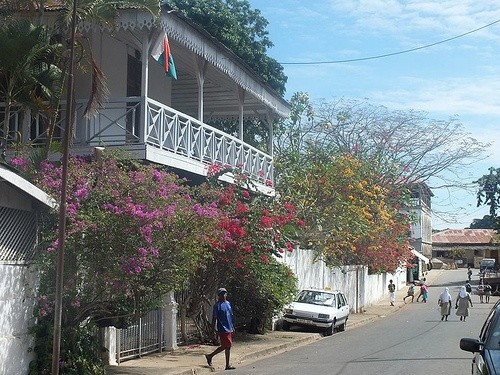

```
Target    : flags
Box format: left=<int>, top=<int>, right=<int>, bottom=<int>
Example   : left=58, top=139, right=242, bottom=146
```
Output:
left=151, top=29, right=177, bottom=80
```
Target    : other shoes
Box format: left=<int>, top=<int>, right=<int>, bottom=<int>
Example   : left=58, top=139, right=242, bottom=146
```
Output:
left=205, top=354, right=214, bottom=366
left=225, top=364, right=237, bottom=370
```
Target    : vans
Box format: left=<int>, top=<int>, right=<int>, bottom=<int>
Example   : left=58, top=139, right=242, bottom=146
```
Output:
left=478, top=258, right=496, bottom=274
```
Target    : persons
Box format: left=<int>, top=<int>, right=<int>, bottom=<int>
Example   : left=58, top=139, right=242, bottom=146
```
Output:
left=403, top=278, right=428, bottom=303
left=205, top=287, right=237, bottom=370
left=465, top=266, right=492, bottom=304
left=438, top=286, right=473, bottom=321
left=388, top=280, right=395, bottom=307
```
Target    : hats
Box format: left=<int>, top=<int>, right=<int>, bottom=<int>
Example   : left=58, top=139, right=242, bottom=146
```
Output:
left=217, top=288, right=228, bottom=295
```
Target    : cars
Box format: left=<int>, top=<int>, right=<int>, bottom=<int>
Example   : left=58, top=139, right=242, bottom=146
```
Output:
left=282, top=288, right=349, bottom=336
left=460, top=298, right=499, bottom=375
